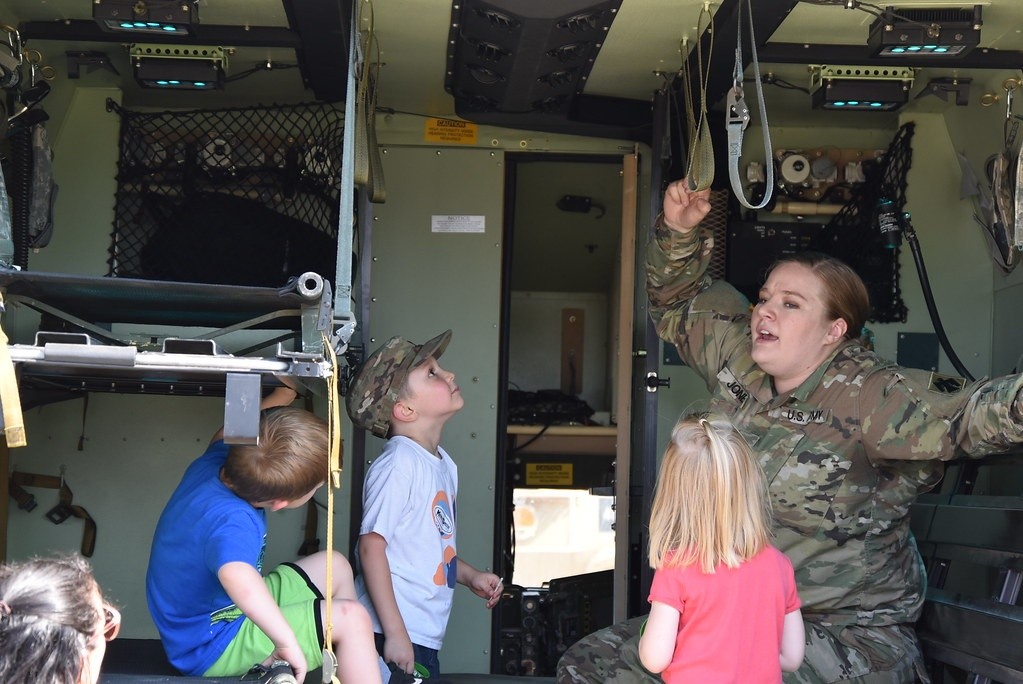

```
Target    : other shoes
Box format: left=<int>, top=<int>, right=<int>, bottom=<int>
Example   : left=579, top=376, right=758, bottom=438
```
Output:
left=264, top=661, right=299, bottom=684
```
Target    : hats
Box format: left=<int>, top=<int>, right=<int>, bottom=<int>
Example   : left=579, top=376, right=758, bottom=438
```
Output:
left=345, top=329, right=454, bottom=439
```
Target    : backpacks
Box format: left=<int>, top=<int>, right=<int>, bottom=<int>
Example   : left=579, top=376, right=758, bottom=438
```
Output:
left=970, top=112, right=1023, bottom=273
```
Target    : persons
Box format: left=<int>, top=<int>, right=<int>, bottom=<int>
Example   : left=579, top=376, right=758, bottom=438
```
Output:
left=0, top=552, right=123, bottom=684
left=638, top=412, right=805, bottom=684
left=345, top=329, right=504, bottom=684
left=145, top=387, right=382, bottom=684
left=556, top=177, right=1023, bottom=684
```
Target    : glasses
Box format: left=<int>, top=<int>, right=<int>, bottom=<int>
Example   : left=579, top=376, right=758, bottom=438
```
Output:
left=85, top=603, right=122, bottom=648
